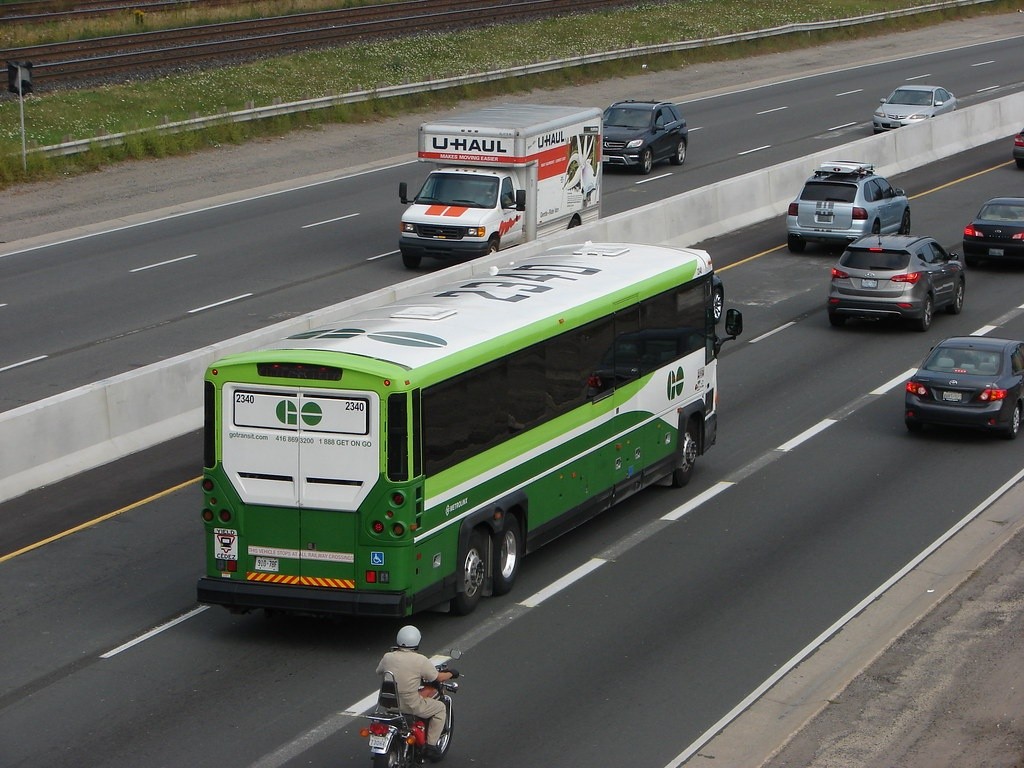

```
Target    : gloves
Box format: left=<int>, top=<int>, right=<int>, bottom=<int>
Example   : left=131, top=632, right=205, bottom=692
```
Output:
left=447, top=669, right=459, bottom=680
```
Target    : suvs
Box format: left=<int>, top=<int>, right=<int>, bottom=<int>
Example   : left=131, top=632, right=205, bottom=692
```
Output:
left=786, top=160, right=911, bottom=253
left=600, top=100, right=688, bottom=174
left=826, top=233, right=965, bottom=333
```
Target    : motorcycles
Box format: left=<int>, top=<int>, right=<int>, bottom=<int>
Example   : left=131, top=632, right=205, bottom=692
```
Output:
left=360, top=648, right=465, bottom=768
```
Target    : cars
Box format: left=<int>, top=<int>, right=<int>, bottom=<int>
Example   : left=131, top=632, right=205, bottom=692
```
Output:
left=906, top=336, right=1024, bottom=438
left=590, top=327, right=706, bottom=394
left=1013, top=128, right=1024, bottom=168
left=963, top=196, right=1024, bottom=269
left=872, top=84, right=957, bottom=134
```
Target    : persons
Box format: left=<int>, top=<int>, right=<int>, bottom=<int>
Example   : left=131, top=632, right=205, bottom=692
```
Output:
left=488, top=185, right=512, bottom=205
left=375, top=625, right=459, bottom=759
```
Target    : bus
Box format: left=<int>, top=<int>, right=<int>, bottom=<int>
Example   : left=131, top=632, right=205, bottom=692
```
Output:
left=196, top=239, right=741, bottom=618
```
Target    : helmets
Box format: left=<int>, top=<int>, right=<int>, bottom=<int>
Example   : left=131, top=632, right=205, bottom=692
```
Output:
left=396, top=624, right=422, bottom=648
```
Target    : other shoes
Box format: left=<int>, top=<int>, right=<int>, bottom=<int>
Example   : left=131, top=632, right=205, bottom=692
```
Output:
left=422, top=745, right=442, bottom=758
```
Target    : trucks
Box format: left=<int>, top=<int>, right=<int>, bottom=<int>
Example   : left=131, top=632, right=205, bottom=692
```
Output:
left=399, top=101, right=602, bottom=269
